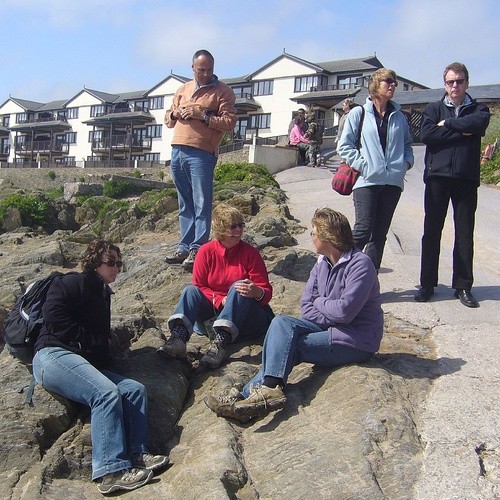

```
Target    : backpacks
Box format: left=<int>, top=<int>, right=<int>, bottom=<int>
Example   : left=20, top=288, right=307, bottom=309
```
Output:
left=2, top=271, right=87, bottom=402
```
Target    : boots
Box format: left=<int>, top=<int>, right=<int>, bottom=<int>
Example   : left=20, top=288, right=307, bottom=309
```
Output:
left=155, top=323, right=191, bottom=361
left=199, top=330, right=231, bottom=369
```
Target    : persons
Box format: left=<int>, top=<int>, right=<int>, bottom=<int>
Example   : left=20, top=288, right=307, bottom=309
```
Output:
left=234, top=208, right=383, bottom=415
left=32, top=239, right=170, bottom=493
left=336, top=68, right=414, bottom=276
left=288, top=108, right=323, bottom=167
left=334, top=99, right=354, bottom=163
left=163, top=202, right=275, bottom=368
left=164, top=50, right=237, bottom=268
left=415, top=62, right=490, bottom=308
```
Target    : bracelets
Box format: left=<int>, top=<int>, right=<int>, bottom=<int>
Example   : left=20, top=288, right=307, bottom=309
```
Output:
left=255, top=286, right=265, bottom=301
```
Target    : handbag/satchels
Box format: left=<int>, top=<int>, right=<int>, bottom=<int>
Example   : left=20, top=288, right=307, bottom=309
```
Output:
left=332, top=161, right=362, bottom=195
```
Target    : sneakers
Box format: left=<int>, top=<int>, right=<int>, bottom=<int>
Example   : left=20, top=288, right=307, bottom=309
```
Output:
left=97, top=466, right=154, bottom=495
left=164, top=251, right=186, bottom=264
left=205, top=387, right=259, bottom=421
left=124, top=452, right=170, bottom=471
left=181, top=248, right=199, bottom=269
left=233, top=382, right=287, bottom=415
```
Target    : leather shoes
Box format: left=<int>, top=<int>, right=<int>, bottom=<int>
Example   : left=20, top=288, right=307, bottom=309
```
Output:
left=414, top=287, right=435, bottom=302
left=454, top=288, right=478, bottom=307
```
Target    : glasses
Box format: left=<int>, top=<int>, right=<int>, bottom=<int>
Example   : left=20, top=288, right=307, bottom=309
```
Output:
left=380, top=78, right=398, bottom=87
left=445, top=79, right=466, bottom=86
left=310, top=231, right=316, bottom=237
left=101, top=260, right=123, bottom=267
left=225, top=222, right=245, bottom=229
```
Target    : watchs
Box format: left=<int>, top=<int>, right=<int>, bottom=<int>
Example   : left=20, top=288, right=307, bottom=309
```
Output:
left=200, top=111, right=210, bottom=123
